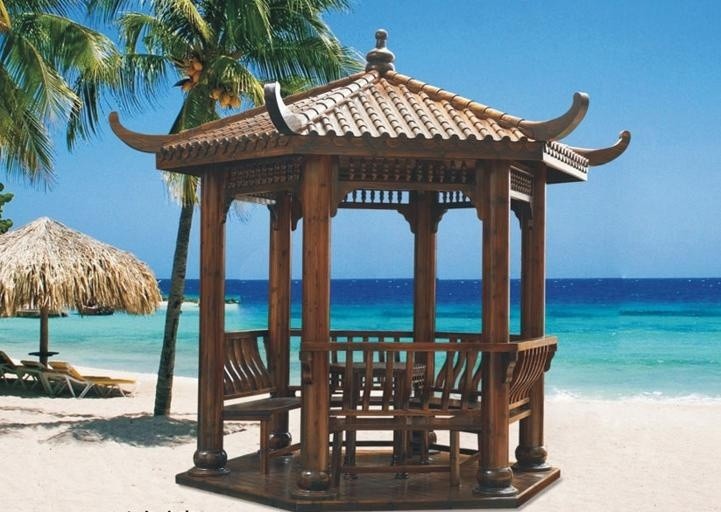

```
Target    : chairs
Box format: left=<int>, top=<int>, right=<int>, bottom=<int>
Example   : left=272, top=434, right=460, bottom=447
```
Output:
left=0, top=351, right=136, bottom=397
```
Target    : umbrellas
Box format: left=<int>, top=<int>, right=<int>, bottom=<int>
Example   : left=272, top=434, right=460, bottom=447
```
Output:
left=0, top=215, right=164, bottom=370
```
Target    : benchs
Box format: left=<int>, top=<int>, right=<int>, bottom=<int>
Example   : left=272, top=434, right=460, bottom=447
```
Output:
left=223, top=331, right=557, bottom=491
